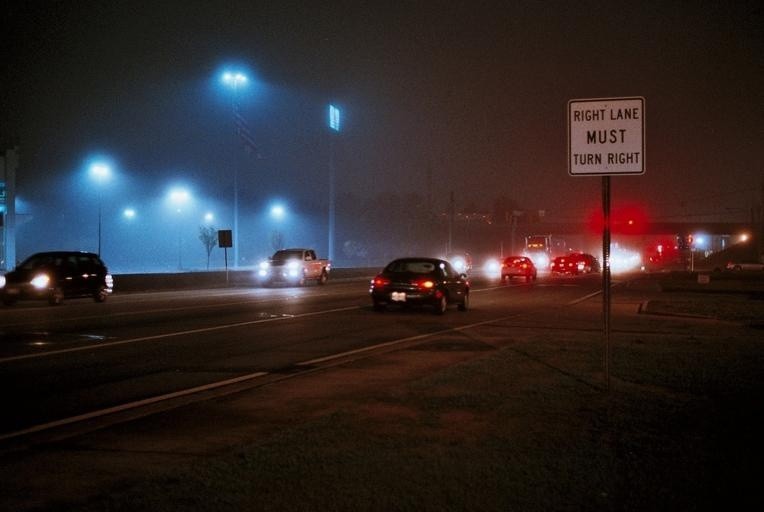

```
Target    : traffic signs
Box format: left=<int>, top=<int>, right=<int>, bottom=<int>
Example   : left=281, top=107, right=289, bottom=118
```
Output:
left=567, top=95, right=646, bottom=177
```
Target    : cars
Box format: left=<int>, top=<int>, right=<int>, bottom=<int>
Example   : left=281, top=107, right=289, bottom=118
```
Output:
left=0, top=251, right=113, bottom=307
left=724, top=256, right=764, bottom=272
left=369, top=253, right=600, bottom=315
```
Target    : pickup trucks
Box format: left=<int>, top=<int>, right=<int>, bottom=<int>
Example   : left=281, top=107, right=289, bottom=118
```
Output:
left=258, top=248, right=332, bottom=286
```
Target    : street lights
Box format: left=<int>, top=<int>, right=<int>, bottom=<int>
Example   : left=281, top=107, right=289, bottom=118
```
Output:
left=89, top=164, right=214, bottom=270
left=272, top=208, right=282, bottom=248
left=220, top=69, right=249, bottom=264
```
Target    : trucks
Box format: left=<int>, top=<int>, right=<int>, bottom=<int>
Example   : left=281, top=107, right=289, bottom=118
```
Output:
left=525, top=234, right=566, bottom=268
